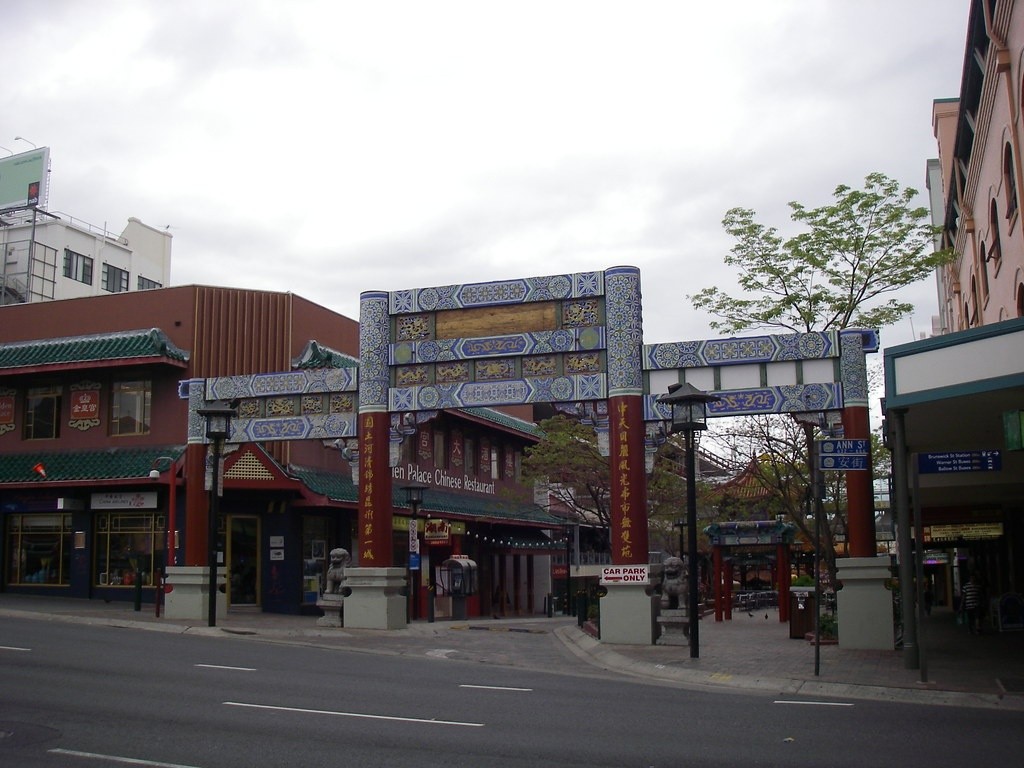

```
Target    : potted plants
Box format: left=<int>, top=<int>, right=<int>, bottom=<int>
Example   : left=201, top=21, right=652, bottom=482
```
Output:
left=819, top=613, right=837, bottom=641
left=789, top=575, right=816, bottom=592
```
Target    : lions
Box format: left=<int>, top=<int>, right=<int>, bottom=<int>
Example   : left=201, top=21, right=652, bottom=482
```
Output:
left=660, top=556, right=687, bottom=610
left=324, top=548, right=352, bottom=595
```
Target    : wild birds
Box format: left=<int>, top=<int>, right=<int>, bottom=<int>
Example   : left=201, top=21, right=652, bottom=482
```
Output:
left=748, top=612, right=755, bottom=619
left=765, top=613, right=768, bottom=620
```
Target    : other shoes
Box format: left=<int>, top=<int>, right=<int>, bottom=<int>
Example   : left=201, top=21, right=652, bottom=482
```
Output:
left=968, top=629, right=972, bottom=634
left=976, top=629, right=982, bottom=634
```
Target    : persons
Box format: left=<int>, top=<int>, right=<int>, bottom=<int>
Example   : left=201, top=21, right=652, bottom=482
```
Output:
left=923, top=578, right=936, bottom=618
left=959, top=572, right=986, bottom=641
